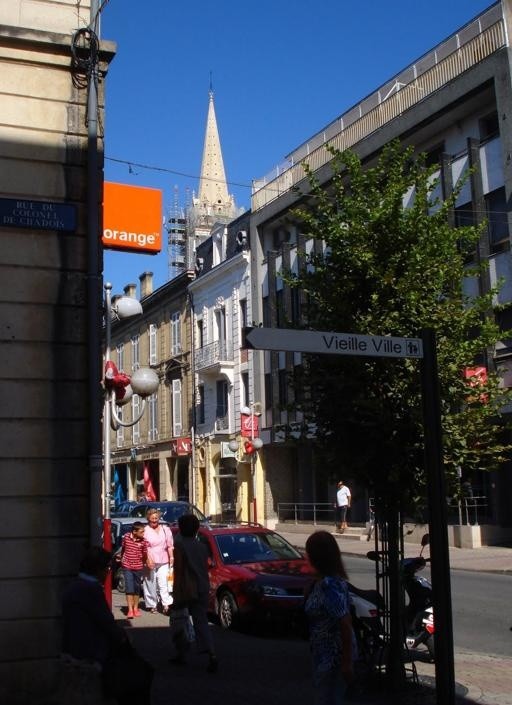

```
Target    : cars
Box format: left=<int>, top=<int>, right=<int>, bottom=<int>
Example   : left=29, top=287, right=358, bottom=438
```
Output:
left=100, top=498, right=328, bottom=637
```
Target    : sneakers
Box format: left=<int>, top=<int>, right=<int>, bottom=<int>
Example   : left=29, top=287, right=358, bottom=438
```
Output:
left=134, top=608, right=142, bottom=616
left=163, top=608, right=169, bottom=615
left=151, top=608, right=157, bottom=613
left=127, top=610, right=134, bottom=618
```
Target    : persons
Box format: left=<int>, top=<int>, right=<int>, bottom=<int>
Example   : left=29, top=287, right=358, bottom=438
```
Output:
left=59, top=544, right=156, bottom=705
left=143, top=508, right=176, bottom=616
left=120, top=521, right=149, bottom=619
left=304, top=531, right=369, bottom=705
left=332, top=480, right=351, bottom=533
left=171, top=514, right=220, bottom=676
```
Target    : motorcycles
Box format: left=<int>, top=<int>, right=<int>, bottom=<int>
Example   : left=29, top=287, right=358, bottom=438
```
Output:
left=346, top=530, right=435, bottom=667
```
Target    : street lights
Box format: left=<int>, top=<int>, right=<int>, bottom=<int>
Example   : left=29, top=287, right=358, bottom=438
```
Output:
left=102, top=281, right=165, bottom=554
left=227, top=400, right=269, bottom=525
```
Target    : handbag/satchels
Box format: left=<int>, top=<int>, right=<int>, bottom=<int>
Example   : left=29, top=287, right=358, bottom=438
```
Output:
left=350, top=604, right=378, bottom=675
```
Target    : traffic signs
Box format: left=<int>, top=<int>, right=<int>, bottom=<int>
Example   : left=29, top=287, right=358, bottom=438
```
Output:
left=242, top=327, right=425, bottom=360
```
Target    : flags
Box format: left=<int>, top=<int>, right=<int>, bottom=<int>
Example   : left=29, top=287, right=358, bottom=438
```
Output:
left=143, top=461, right=157, bottom=502
left=113, top=465, right=127, bottom=512
